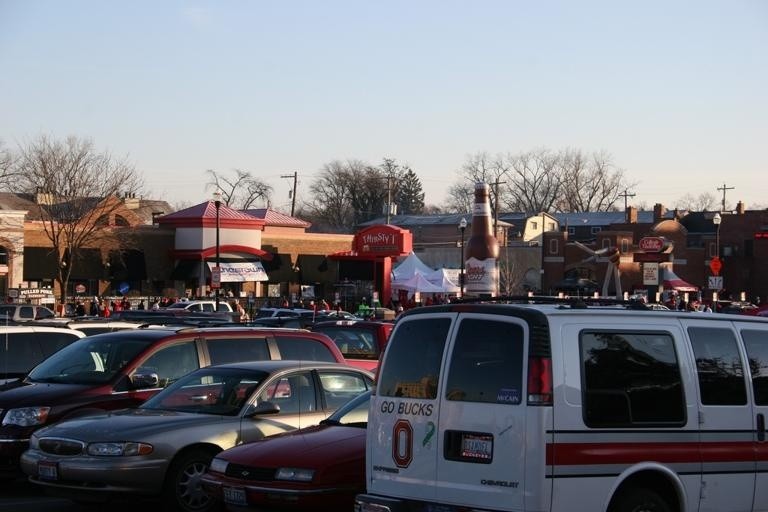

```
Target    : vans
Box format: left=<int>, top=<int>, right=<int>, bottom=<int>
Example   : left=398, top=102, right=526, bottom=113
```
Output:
left=0, top=323, right=348, bottom=461
left=333, top=283, right=358, bottom=299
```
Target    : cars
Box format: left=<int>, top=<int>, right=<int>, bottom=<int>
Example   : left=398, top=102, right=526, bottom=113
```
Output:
left=200, top=388, right=372, bottom=511
left=0, top=299, right=767, bottom=370
left=552, top=279, right=599, bottom=298
left=20, top=359, right=377, bottom=511
left=0, top=321, right=105, bottom=387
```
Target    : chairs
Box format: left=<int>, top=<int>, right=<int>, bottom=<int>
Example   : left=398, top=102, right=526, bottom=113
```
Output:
left=280, top=384, right=313, bottom=414
left=235, top=383, right=266, bottom=412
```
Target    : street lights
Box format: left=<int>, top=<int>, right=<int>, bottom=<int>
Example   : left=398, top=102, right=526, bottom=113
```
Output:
left=214, top=187, right=222, bottom=312
left=713, top=212, right=722, bottom=313
left=106, top=262, right=110, bottom=297
left=293, top=266, right=302, bottom=301
left=460, top=218, right=467, bottom=299
left=60, top=262, right=66, bottom=304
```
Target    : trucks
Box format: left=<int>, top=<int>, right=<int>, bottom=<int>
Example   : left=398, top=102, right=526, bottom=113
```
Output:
left=353, top=293, right=767, bottom=511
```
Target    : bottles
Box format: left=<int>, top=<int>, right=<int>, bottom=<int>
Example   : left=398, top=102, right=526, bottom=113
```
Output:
left=460, top=183, right=503, bottom=300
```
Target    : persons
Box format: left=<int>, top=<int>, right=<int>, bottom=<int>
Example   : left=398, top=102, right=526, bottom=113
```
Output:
left=25, top=294, right=31, bottom=305
left=395, top=292, right=457, bottom=313
left=227, top=288, right=342, bottom=323
left=664, top=294, right=712, bottom=314
left=74, top=291, right=191, bottom=318
